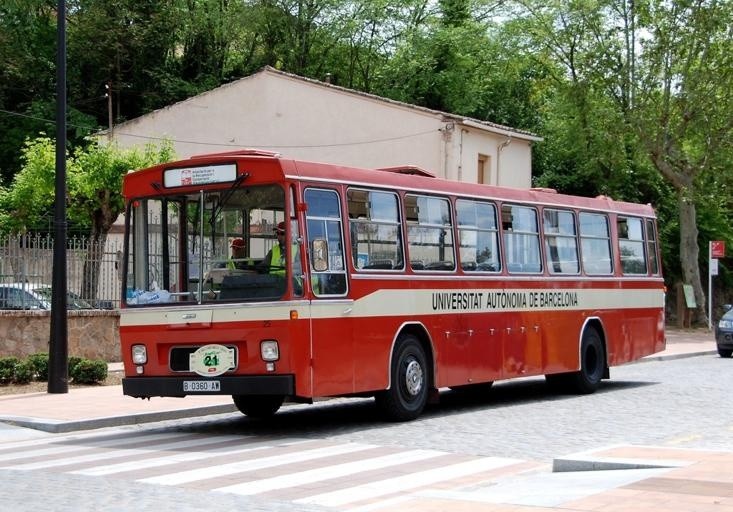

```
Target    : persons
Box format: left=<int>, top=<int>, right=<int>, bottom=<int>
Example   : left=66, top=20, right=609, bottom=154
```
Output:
left=258, top=221, right=320, bottom=295
left=211, top=238, right=254, bottom=289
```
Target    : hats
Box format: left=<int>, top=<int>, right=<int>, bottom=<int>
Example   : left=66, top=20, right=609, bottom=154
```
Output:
left=272, top=222, right=285, bottom=232
left=230, top=238, right=245, bottom=249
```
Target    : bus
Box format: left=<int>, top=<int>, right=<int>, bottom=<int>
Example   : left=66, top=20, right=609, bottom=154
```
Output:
left=118, top=149, right=669, bottom=420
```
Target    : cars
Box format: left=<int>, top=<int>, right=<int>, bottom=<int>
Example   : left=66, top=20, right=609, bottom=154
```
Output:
left=714, top=304, right=733, bottom=357
left=0, top=283, right=106, bottom=310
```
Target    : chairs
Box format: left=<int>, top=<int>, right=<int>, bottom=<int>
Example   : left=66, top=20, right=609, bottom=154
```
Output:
left=363, top=257, right=653, bottom=275
